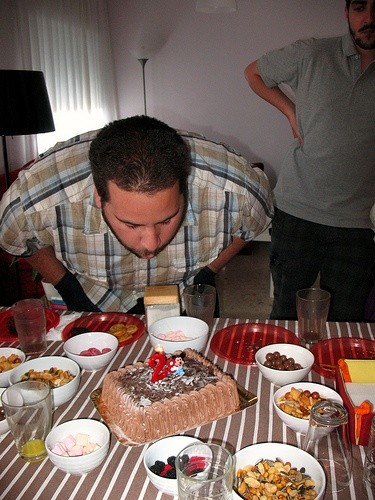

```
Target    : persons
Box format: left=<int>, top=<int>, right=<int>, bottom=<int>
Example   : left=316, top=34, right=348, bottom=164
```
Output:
left=244, top=0, right=375, bottom=323
left=0, top=114, right=276, bottom=317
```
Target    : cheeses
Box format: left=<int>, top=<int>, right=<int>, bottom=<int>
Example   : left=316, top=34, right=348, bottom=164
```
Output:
left=52, top=434, right=100, bottom=457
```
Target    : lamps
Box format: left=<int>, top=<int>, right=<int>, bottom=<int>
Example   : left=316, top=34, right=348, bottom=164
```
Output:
left=0, top=69, right=55, bottom=173
left=126, top=21, right=164, bottom=116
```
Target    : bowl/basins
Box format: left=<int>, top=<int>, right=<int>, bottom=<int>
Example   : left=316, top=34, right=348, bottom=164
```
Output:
left=254, top=342, right=315, bottom=386
left=142, top=437, right=214, bottom=495
left=44, top=419, right=111, bottom=476
left=0, top=327, right=120, bottom=438
left=148, top=316, right=209, bottom=353
left=222, top=442, right=326, bottom=500
left=272, top=381, right=344, bottom=436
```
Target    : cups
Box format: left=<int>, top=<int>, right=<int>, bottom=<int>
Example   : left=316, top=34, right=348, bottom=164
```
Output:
left=294, top=289, right=331, bottom=343
left=3, top=380, right=54, bottom=463
left=175, top=442, right=234, bottom=500
left=12, top=297, right=47, bottom=355
left=182, top=285, right=216, bottom=333
left=303, top=403, right=353, bottom=494
left=363, top=418, right=375, bottom=488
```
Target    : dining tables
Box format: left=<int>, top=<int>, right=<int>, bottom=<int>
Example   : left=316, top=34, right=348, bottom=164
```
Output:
left=0, top=306, right=375, bottom=500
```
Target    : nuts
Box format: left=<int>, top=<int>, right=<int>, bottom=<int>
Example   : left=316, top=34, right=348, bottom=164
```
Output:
left=236, top=462, right=316, bottom=500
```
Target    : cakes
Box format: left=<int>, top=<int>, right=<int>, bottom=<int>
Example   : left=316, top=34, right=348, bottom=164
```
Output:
left=100, top=347, right=240, bottom=443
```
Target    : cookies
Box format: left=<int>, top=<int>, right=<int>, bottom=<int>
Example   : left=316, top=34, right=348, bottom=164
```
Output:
left=105, top=324, right=137, bottom=342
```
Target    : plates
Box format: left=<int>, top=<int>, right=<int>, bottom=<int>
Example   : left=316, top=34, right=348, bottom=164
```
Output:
left=0, top=304, right=60, bottom=344
left=63, top=312, right=145, bottom=349
left=309, top=337, right=375, bottom=379
left=209, top=323, right=300, bottom=366
